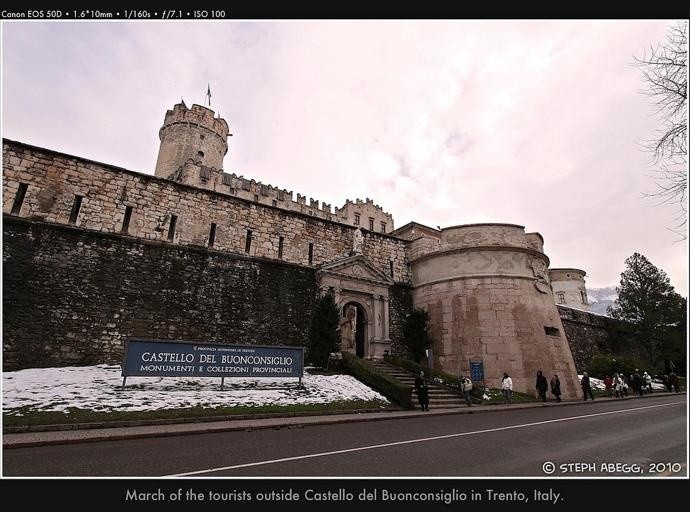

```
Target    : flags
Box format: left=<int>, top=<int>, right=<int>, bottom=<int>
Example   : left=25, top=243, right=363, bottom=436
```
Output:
left=208, top=83, right=211, bottom=106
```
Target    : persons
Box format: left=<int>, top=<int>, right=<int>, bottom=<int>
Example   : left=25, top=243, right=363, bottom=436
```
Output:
left=501, top=373, right=513, bottom=406
left=353, top=226, right=365, bottom=253
left=415, top=370, right=429, bottom=411
left=604, top=369, right=681, bottom=399
left=536, top=370, right=548, bottom=404
left=550, top=375, right=561, bottom=403
left=460, top=374, right=473, bottom=405
left=581, top=372, right=594, bottom=401
